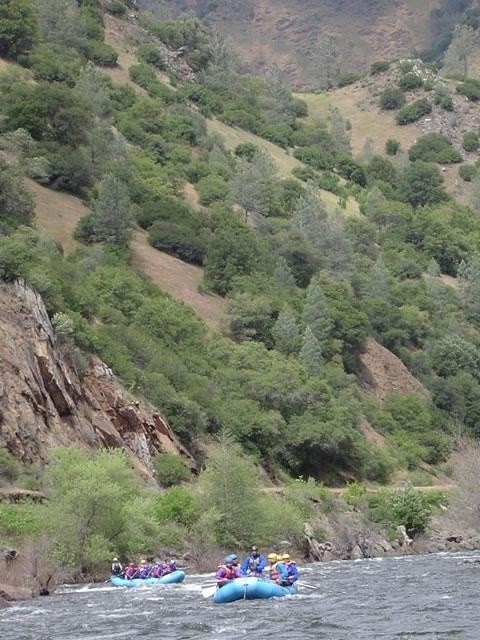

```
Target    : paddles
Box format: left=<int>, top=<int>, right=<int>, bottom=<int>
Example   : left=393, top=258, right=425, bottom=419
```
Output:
left=201, top=580, right=217, bottom=600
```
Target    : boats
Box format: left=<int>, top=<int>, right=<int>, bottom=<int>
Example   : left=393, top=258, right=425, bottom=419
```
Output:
left=213, top=575, right=297, bottom=603
left=110, top=569, right=185, bottom=589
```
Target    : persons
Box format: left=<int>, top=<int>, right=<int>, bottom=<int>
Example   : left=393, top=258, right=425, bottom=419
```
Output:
left=262, top=552, right=300, bottom=586
left=215, top=556, right=244, bottom=589
left=228, top=553, right=244, bottom=571
left=240, top=545, right=268, bottom=577
left=110, top=556, right=178, bottom=580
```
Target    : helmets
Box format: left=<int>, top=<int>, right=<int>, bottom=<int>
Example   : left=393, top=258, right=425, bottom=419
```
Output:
left=229, top=553, right=238, bottom=560
left=224, top=557, right=232, bottom=563
left=251, top=545, right=258, bottom=551
left=267, top=553, right=290, bottom=563
